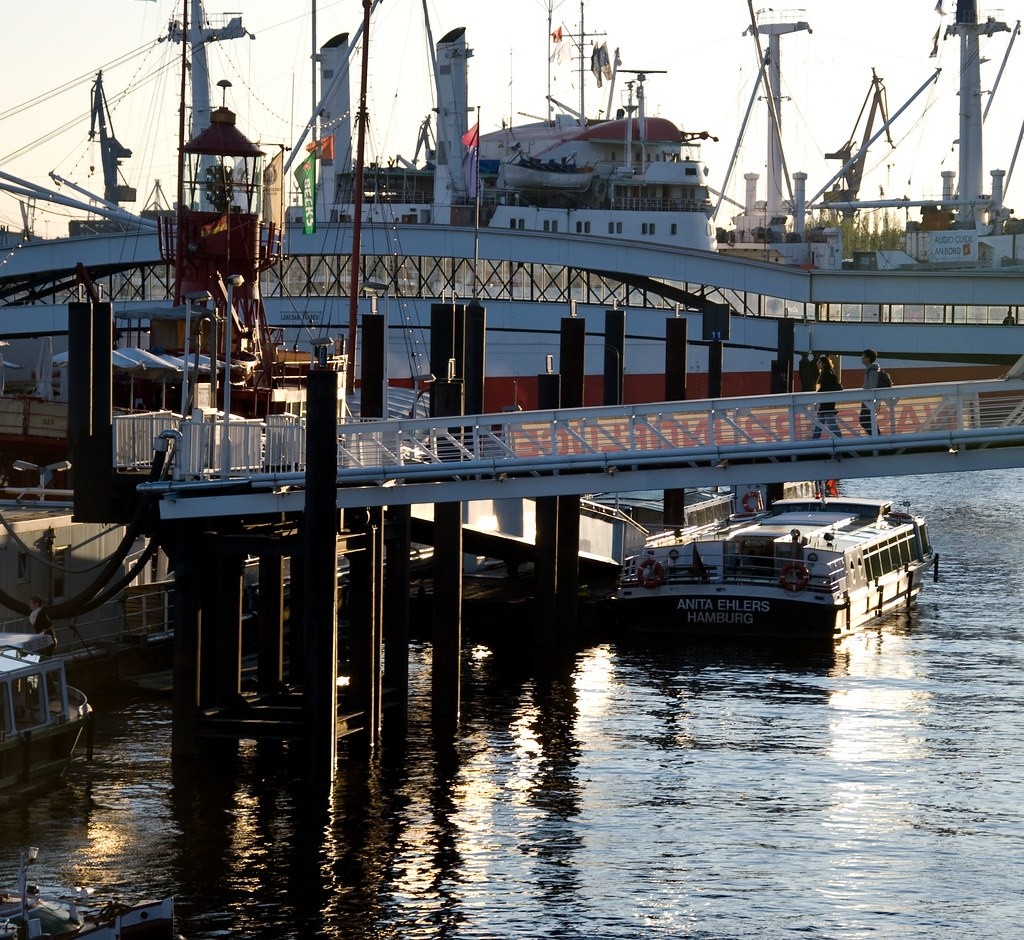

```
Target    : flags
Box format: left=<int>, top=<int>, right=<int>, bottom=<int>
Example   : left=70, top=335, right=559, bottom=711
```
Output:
left=305, top=134, right=335, bottom=160
left=294, top=149, right=316, bottom=235
left=591, top=39, right=613, bottom=88
left=264, top=149, right=286, bottom=236
left=461, top=122, right=480, bottom=148
left=548, top=26, right=575, bottom=68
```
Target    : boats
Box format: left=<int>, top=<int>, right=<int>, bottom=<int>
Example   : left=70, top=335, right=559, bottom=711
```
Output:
left=0, top=847, right=173, bottom=940
left=1, top=630, right=92, bottom=798
left=1, top=1, right=1023, bottom=460
left=617, top=492, right=939, bottom=640
left=729, top=479, right=838, bottom=513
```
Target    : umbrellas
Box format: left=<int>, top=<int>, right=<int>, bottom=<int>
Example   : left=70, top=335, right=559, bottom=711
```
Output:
left=34, top=336, right=54, bottom=400
left=50, top=344, right=244, bottom=414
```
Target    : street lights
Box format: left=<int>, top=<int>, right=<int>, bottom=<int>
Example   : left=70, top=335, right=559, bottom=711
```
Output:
left=221, top=274, right=245, bottom=474
left=180, top=290, right=212, bottom=418
left=13, top=459, right=71, bottom=502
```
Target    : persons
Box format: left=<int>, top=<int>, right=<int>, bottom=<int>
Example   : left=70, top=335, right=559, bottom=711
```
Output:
left=812, top=358, right=843, bottom=438
left=28, top=594, right=59, bottom=700
left=859, top=350, right=884, bottom=436
left=1003, top=312, right=1014, bottom=325
left=799, top=350, right=819, bottom=392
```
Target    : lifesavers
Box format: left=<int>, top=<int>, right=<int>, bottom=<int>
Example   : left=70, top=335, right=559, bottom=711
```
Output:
left=638, top=560, right=664, bottom=588
left=743, top=491, right=764, bottom=512
left=780, top=561, right=808, bottom=592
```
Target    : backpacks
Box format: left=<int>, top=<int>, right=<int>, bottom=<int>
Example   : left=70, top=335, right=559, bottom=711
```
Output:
left=867, top=368, right=892, bottom=388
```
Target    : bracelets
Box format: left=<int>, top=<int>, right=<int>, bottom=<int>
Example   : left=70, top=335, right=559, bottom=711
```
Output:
left=41, top=631, right=45, bottom=635
